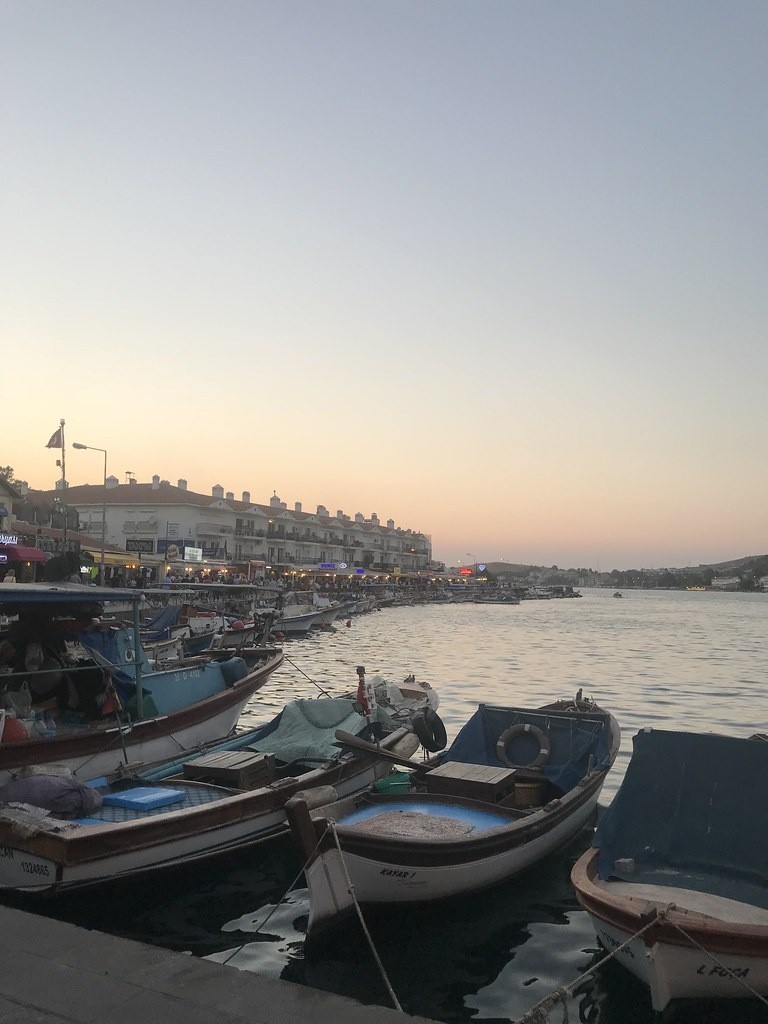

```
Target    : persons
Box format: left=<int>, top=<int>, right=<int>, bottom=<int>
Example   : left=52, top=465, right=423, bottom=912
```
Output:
left=3, top=569, right=16, bottom=583
left=70, top=570, right=431, bottom=606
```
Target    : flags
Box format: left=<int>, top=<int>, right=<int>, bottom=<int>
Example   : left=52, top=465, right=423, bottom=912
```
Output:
left=44, top=428, right=62, bottom=449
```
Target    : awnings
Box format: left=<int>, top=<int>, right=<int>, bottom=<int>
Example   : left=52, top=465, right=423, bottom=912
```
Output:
left=88, top=552, right=139, bottom=566
left=7, top=545, right=48, bottom=562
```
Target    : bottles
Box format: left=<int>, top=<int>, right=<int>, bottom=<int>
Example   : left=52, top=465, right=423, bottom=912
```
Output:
left=36, top=716, right=48, bottom=738
left=46, top=715, right=56, bottom=737
left=5, top=705, right=16, bottom=719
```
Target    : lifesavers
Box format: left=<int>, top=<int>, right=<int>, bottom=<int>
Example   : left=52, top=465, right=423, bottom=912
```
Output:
left=497, top=723, right=550, bottom=768
left=413, top=706, right=446, bottom=752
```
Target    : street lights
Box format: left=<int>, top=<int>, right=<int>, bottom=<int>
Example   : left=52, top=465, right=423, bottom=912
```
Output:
left=72, top=443, right=108, bottom=588
left=268, top=517, right=287, bottom=590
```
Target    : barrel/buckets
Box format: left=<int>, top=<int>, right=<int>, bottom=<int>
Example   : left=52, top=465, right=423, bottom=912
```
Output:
left=375, top=773, right=411, bottom=793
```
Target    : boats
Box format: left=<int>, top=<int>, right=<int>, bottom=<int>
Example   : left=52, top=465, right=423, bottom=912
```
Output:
left=283, top=687, right=620, bottom=937
left=114, top=587, right=583, bottom=664
left=568, top=726, right=768, bottom=1012
left=0, top=581, right=285, bottom=788
left=0, top=674, right=440, bottom=897
